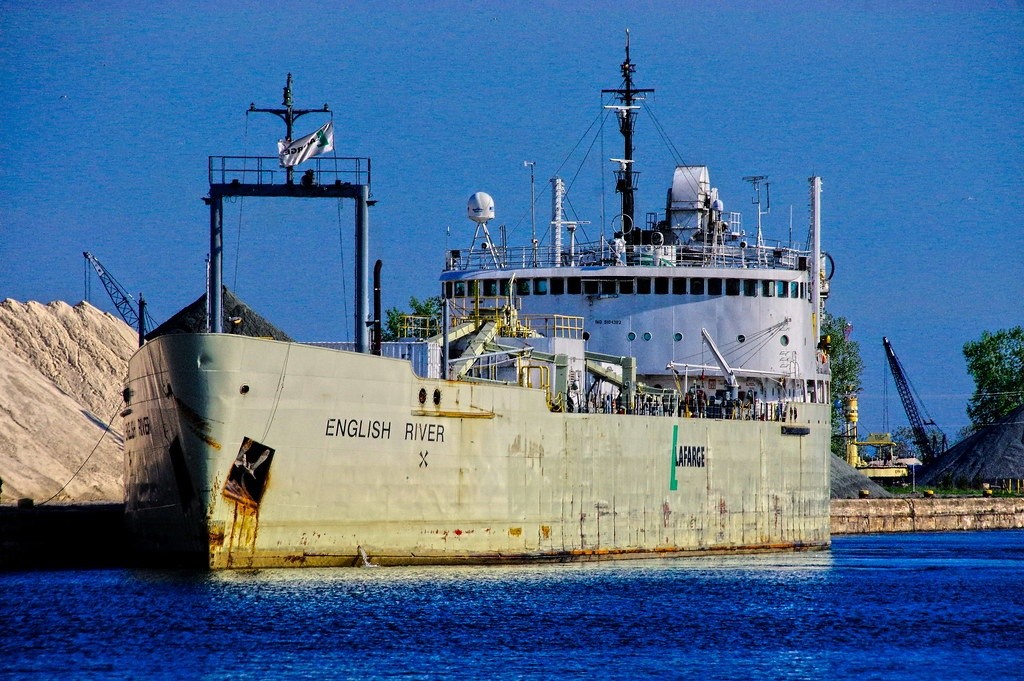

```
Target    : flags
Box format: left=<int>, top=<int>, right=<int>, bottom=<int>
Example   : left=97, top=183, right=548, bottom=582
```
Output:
left=276, top=121, right=334, bottom=168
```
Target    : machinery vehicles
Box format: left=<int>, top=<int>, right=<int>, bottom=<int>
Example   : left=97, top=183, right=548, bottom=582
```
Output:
left=83, top=252, right=159, bottom=336
left=882, top=337, right=948, bottom=464
left=838, top=384, right=909, bottom=485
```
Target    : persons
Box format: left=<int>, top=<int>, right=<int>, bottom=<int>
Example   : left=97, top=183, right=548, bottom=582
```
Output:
left=567, top=383, right=708, bottom=421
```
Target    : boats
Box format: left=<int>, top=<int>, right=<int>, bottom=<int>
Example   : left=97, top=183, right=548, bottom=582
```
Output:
left=122, top=29, right=835, bottom=572
